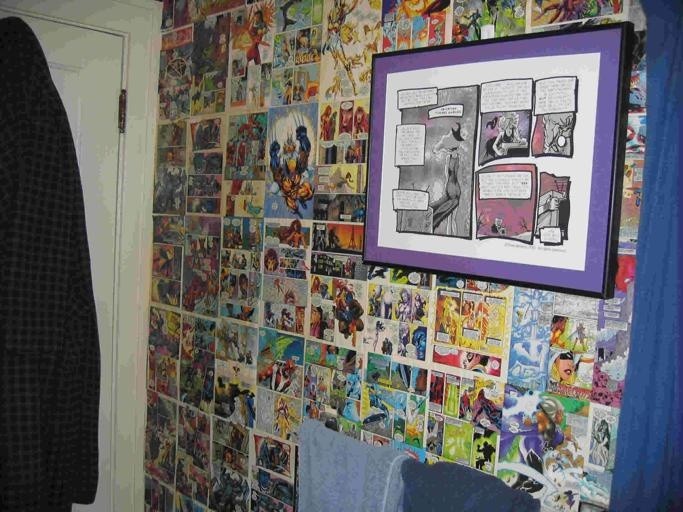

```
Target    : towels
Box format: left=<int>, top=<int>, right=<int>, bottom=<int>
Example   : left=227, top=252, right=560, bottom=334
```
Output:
left=299, top=419, right=408, bottom=512
left=401, top=459, right=540, bottom=512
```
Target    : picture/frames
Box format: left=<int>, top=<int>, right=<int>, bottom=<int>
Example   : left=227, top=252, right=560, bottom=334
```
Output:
left=363, top=22, right=634, bottom=299
